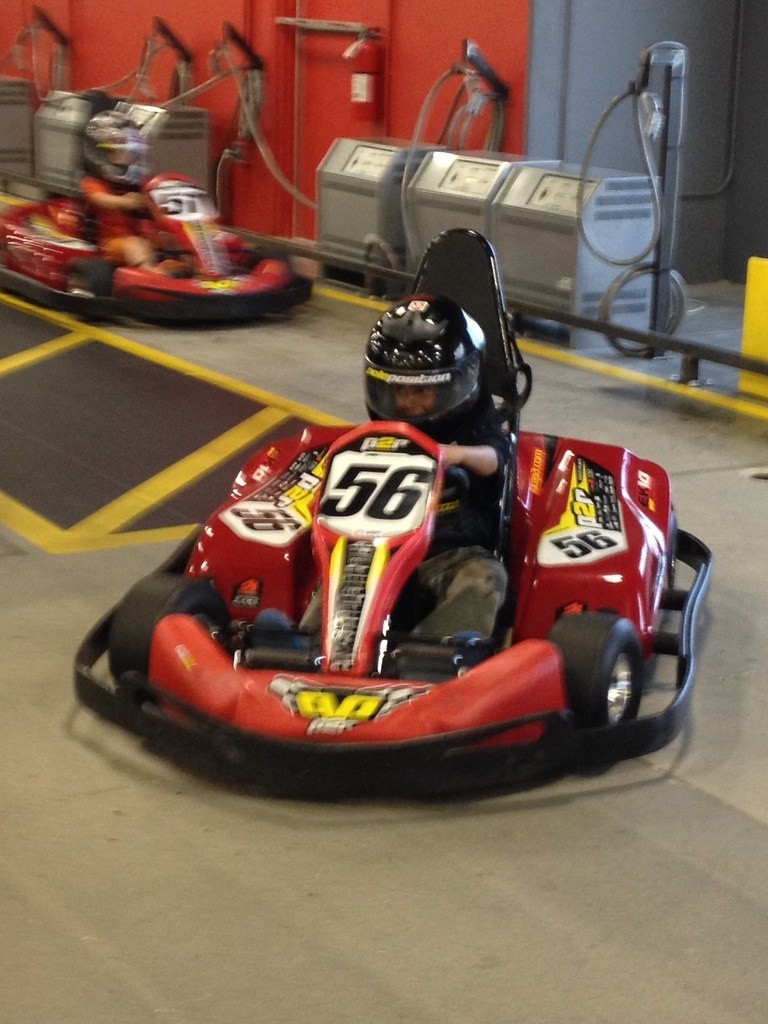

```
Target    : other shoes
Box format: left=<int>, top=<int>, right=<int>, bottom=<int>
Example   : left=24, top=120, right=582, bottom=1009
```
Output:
left=253, top=609, right=302, bottom=652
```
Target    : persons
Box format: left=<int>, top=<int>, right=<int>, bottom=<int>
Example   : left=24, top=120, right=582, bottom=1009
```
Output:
left=250, top=295, right=510, bottom=683
left=78, top=111, right=269, bottom=276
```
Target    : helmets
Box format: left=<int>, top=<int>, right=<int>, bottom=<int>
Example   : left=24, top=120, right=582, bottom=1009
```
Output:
left=362, top=292, right=487, bottom=438
left=82, top=109, right=147, bottom=187
